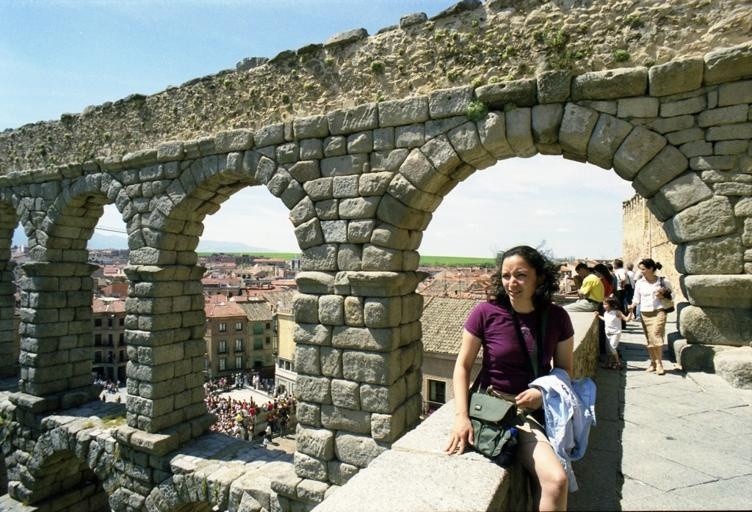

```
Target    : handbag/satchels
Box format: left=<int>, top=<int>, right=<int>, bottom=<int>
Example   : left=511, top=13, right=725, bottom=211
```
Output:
left=661, top=293, right=675, bottom=313
left=461, top=386, right=520, bottom=465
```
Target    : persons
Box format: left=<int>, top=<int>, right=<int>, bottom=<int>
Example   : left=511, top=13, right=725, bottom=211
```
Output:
left=444, top=242, right=597, bottom=512
left=201, top=368, right=296, bottom=443
left=92, top=373, right=122, bottom=404
left=562, top=256, right=675, bottom=375
